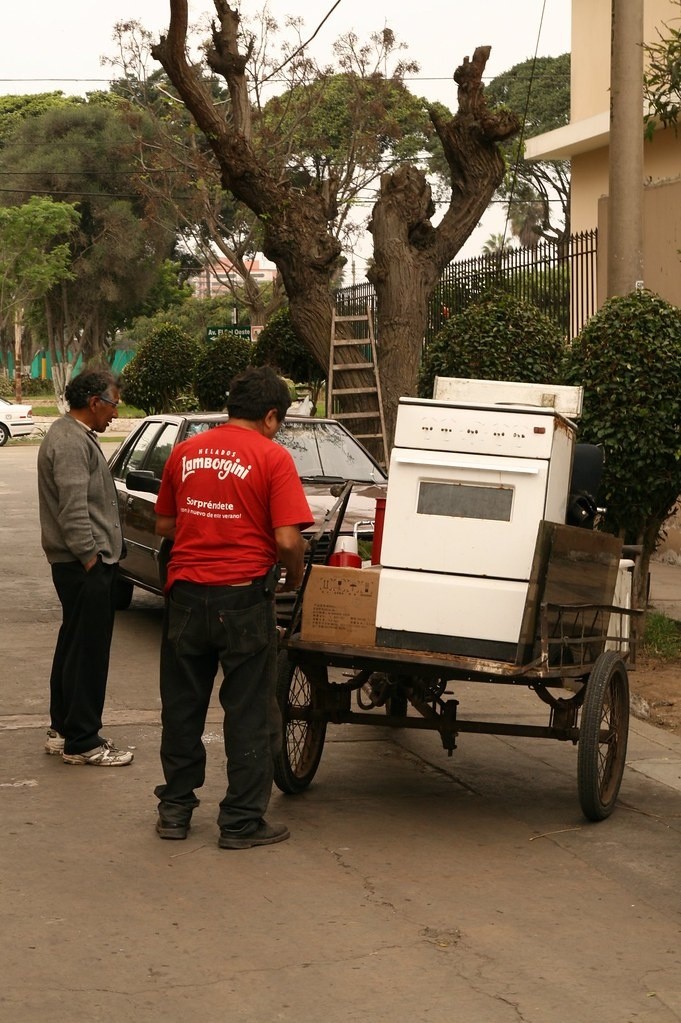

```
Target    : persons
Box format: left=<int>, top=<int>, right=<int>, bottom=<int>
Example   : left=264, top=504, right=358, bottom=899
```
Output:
left=38, top=369, right=134, bottom=767
left=154, top=369, right=316, bottom=848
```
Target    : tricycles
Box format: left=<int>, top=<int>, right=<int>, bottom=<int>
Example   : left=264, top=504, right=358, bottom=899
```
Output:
left=264, top=481, right=652, bottom=824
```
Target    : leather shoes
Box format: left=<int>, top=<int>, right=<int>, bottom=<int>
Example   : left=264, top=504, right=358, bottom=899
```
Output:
left=218, top=818, right=290, bottom=848
left=155, top=818, right=190, bottom=839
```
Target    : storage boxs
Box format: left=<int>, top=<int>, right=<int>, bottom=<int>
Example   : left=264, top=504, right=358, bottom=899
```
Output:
left=299, top=564, right=382, bottom=648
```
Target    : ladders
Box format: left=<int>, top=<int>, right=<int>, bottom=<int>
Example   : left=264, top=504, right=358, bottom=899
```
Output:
left=326, top=305, right=390, bottom=476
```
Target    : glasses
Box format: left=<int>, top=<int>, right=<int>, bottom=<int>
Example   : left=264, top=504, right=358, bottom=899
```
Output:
left=88, top=391, right=120, bottom=410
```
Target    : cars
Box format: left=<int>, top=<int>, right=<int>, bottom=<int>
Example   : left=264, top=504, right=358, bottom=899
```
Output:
left=0, top=397, right=36, bottom=447
left=103, top=410, right=391, bottom=635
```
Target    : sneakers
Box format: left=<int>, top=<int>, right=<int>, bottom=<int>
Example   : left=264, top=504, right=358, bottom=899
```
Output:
left=45, top=728, right=114, bottom=754
left=62, top=742, right=134, bottom=766
